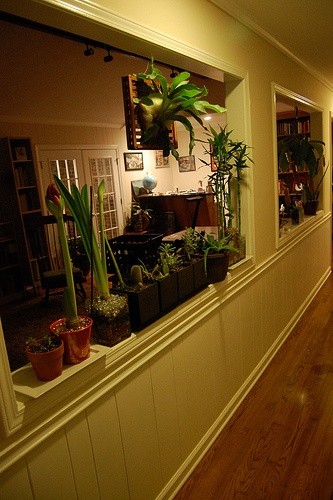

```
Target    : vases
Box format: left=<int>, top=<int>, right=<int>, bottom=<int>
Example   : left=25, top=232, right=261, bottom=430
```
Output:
left=49, top=315, right=93, bottom=365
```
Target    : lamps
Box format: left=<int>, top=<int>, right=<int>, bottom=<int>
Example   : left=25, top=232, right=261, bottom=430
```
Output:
left=170, top=69, right=178, bottom=79
left=83, top=44, right=94, bottom=57
left=103, top=50, right=113, bottom=63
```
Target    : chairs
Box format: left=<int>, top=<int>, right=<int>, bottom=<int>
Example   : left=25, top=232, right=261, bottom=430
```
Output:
left=30, top=214, right=87, bottom=302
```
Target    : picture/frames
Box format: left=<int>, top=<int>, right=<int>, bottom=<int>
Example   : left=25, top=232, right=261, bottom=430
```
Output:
left=179, top=155, right=196, bottom=172
left=124, top=152, right=144, bottom=171
left=210, top=144, right=218, bottom=172
left=155, top=150, right=171, bottom=168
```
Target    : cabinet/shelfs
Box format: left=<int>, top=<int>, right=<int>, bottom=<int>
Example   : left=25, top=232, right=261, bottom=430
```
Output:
left=0, top=136, right=56, bottom=308
left=276, top=109, right=311, bottom=206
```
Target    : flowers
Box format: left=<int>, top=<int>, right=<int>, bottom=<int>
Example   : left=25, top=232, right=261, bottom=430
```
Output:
left=44, top=182, right=82, bottom=328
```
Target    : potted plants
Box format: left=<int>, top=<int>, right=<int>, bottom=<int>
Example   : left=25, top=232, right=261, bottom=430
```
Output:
left=277, top=134, right=329, bottom=225
left=21, top=55, right=255, bottom=383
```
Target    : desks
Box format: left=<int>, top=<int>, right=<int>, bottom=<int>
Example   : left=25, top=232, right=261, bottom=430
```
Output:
left=137, top=191, right=223, bottom=230
